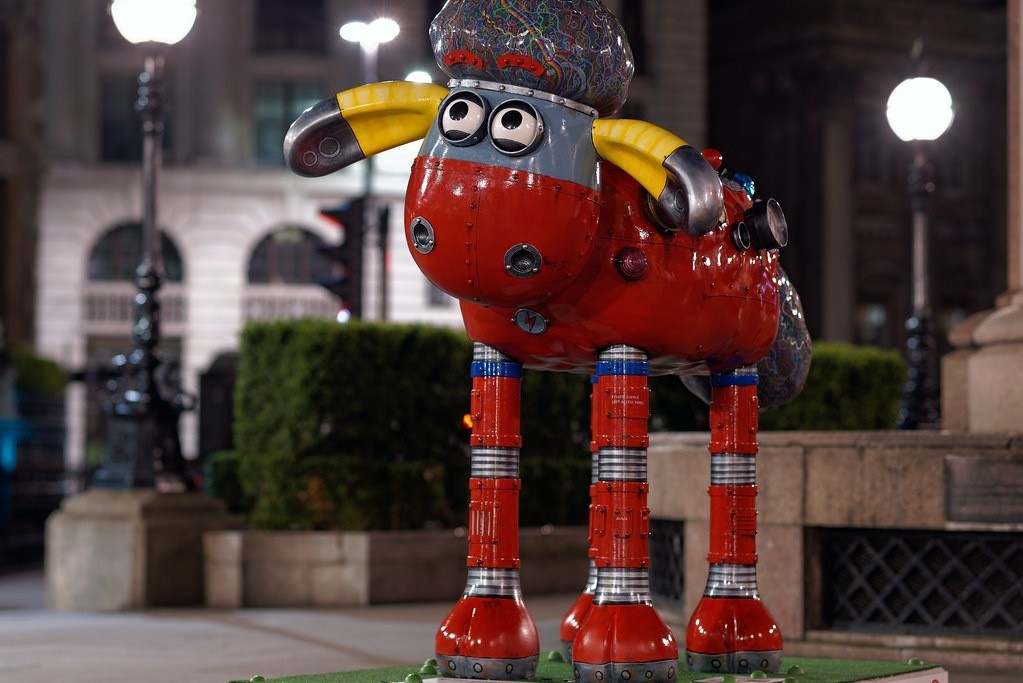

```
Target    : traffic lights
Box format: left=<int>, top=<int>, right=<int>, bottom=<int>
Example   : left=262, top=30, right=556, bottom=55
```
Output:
left=310, top=195, right=367, bottom=321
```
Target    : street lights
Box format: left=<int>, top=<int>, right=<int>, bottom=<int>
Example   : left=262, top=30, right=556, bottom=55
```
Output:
left=338, top=12, right=401, bottom=322
left=883, top=76, right=957, bottom=429
left=84, top=0, right=204, bottom=493
